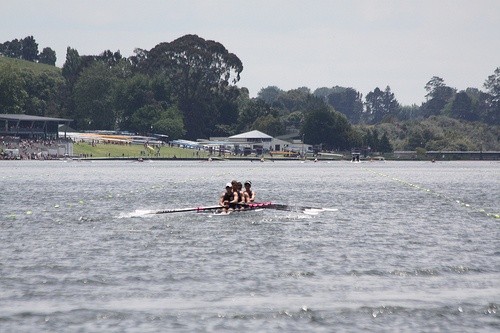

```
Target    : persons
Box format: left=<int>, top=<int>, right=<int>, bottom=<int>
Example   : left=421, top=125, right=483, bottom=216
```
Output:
left=232, top=180, right=255, bottom=203
left=219, top=183, right=238, bottom=214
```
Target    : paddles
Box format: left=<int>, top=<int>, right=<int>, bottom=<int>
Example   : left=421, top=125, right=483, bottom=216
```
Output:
left=129, top=205, right=229, bottom=217
left=229, top=202, right=322, bottom=212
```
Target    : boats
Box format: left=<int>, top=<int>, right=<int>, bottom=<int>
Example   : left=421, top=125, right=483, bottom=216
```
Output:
left=210, top=208, right=264, bottom=216
left=351, top=153, right=361, bottom=163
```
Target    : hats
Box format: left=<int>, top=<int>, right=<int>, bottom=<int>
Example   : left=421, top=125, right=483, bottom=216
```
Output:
left=244, top=180, right=251, bottom=186
left=225, top=182, right=232, bottom=188
